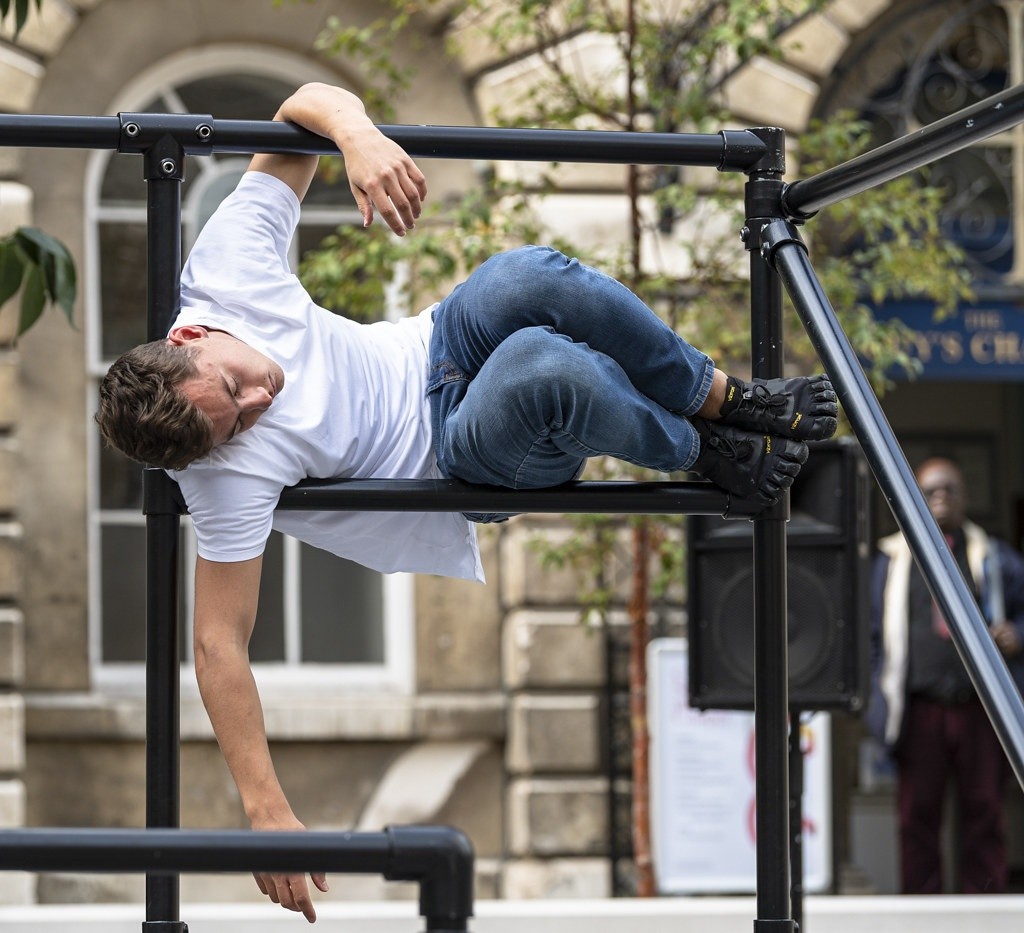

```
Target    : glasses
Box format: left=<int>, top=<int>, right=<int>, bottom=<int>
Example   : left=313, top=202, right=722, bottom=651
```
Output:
left=920, top=476, right=968, bottom=502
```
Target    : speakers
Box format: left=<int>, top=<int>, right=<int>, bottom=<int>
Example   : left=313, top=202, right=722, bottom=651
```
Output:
left=685, top=434, right=866, bottom=711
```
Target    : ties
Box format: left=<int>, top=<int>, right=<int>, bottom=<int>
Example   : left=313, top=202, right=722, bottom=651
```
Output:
left=931, top=532, right=953, bottom=638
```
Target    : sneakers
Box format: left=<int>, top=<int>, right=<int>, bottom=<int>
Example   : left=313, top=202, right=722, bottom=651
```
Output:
left=712, top=372, right=839, bottom=442
left=688, top=414, right=808, bottom=508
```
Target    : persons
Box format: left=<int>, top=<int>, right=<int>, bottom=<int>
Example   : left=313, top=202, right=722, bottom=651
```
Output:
left=860, top=458, right=1024, bottom=897
left=92, top=82, right=841, bottom=925
